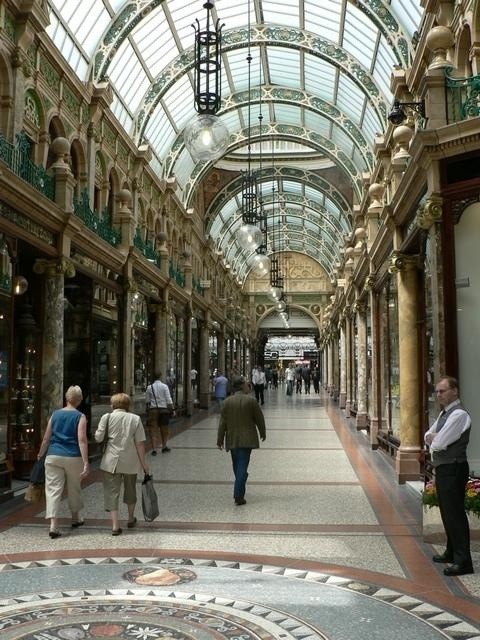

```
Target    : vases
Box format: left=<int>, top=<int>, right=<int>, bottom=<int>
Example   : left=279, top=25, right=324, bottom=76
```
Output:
left=422, top=504, right=480, bottom=555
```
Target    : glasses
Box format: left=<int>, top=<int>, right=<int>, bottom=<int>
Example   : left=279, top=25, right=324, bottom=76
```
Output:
left=434, top=387, right=453, bottom=394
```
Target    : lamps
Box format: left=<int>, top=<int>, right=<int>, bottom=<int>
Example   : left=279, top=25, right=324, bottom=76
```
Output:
left=181, top=0, right=230, bottom=164
left=233, top=1, right=267, bottom=254
left=255, top=43, right=298, bottom=342
left=387, top=98, right=430, bottom=133
left=8, top=273, right=29, bottom=301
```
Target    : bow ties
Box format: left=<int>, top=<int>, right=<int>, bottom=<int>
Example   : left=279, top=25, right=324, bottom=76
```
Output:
left=440, top=410, right=446, bottom=418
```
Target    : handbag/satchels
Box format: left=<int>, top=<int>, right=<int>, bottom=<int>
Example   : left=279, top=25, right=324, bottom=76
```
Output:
left=24, top=476, right=47, bottom=504
left=140, top=475, right=158, bottom=523
left=158, top=409, right=170, bottom=426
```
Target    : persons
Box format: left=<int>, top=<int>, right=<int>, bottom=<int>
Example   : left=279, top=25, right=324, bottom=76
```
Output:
left=213, top=367, right=266, bottom=506
left=37, top=385, right=90, bottom=538
left=136, top=365, right=218, bottom=402
left=145, top=371, right=175, bottom=455
left=251, top=360, right=320, bottom=406
left=94, top=392, right=150, bottom=536
left=423, top=374, right=476, bottom=576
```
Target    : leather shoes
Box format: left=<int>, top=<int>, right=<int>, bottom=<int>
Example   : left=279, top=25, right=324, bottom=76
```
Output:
left=161, top=447, right=171, bottom=452
left=49, top=529, right=62, bottom=539
left=151, top=450, right=157, bottom=456
left=432, top=551, right=453, bottom=563
left=71, top=518, right=85, bottom=527
left=443, top=563, right=475, bottom=577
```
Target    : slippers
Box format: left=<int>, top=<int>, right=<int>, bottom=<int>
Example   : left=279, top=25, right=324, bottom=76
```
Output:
left=112, top=527, right=123, bottom=536
left=127, top=516, right=138, bottom=527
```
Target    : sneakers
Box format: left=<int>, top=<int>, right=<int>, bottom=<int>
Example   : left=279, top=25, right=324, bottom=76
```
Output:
left=233, top=498, right=246, bottom=505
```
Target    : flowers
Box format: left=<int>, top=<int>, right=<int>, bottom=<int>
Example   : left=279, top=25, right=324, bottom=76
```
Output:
left=420, top=477, right=480, bottom=520
